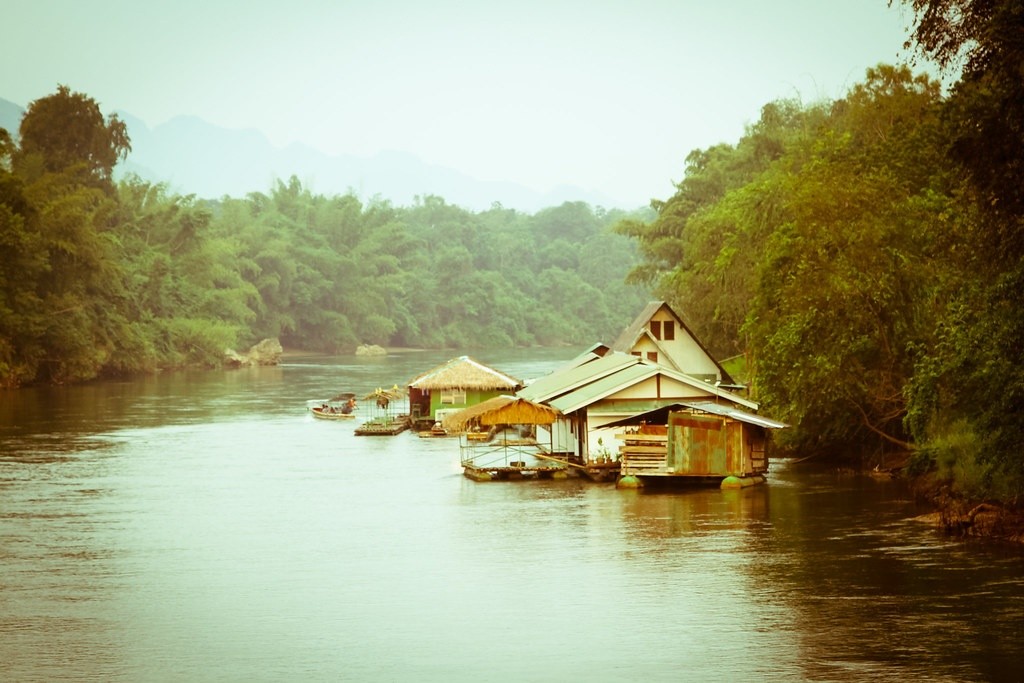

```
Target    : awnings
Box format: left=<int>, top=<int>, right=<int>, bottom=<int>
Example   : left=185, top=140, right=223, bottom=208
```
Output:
left=361, top=388, right=410, bottom=428
left=441, top=395, right=561, bottom=467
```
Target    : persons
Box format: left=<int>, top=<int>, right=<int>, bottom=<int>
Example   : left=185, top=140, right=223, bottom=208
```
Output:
left=322, top=399, right=357, bottom=414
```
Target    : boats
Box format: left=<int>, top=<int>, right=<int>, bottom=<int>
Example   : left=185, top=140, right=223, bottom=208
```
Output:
left=354, top=383, right=411, bottom=435
left=432, top=422, right=446, bottom=435
left=312, top=407, right=355, bottom=419
